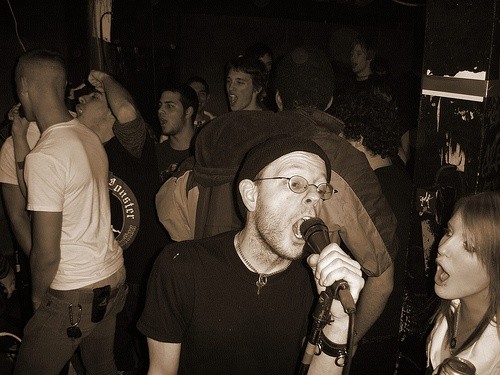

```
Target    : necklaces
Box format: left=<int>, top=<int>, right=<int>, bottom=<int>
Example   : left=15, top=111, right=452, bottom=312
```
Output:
left=450, top=300, right=462, bottom=349
left=235, top=230, right=286, bottom=294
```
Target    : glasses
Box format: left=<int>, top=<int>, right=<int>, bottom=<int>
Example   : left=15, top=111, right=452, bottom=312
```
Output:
left=253, top=175, right=338, bottom=201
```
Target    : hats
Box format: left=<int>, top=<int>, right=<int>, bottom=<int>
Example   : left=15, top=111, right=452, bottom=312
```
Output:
left=236, top=134, right=331, bottom=223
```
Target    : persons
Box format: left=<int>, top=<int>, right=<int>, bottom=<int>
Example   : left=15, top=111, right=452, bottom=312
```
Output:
left=138, top=80, right=207, bottom=307
left=1, top=201, right=34, bottom=374
left=322, top=85, right=430, bottom=375
left=424, top=191, right=500, bottom=375
left=0, top=48, right=125, bottom=375
left=332, top=35, right=412, bottom=167
left=135, top=132, right=365, bottom=375
left=1, top=95, right=80, bottom=262
left=223, top=55, right=270, bottom=114
left=75, top=68, right=151, bottom=375
left=156, top=43, right=400, bottom=373
left=159, top=77, right=219, bottom=143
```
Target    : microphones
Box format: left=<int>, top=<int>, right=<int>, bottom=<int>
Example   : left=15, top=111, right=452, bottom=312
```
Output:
left=301, top=218, right=357, bottom=314
left=18, top=105, right=26, bottom=118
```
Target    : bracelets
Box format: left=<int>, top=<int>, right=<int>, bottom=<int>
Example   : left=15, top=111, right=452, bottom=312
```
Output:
left=316, top=331, right=350, bottom=368
